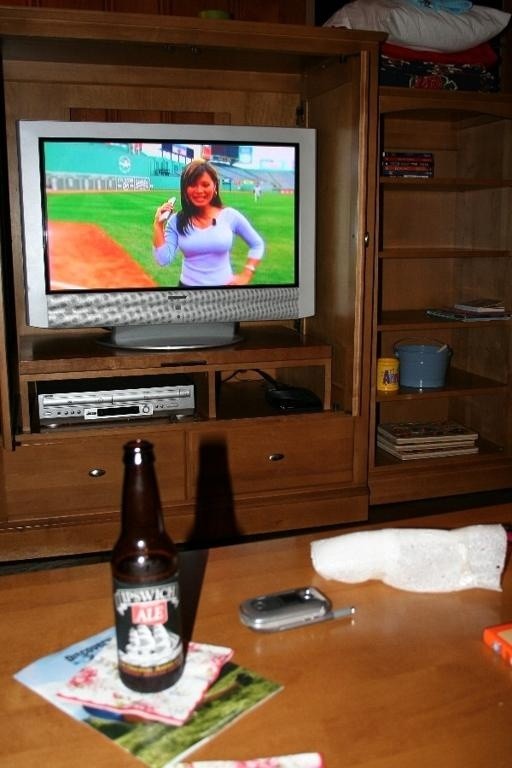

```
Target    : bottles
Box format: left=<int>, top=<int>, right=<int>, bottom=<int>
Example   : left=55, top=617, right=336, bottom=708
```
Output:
left=376, top=357, right=400, bottom=392
left=110, top=438, right=187, bottom=691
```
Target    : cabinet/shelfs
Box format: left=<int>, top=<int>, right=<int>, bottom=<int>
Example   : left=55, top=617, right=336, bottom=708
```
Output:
left=0, top=6, right=390, bottom=564
left=369, top=85, right=512, bottom=505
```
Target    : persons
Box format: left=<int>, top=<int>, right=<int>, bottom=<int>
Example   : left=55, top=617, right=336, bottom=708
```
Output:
left=150, top=156, right=268, bottom=289
left=251, top=181, right=264, bottom=203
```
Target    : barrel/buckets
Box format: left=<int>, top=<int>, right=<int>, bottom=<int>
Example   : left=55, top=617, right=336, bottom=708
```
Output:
left=394, top=334, right=452, bottom=390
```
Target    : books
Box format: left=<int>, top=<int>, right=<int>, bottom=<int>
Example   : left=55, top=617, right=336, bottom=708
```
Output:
left=382, top=162, right=433, bottom=166
left=377, top=440, right=481, bottom=461
left=383, top=156, right=433, bottom=161
left=481, top=621, right=511, bottom=670
left=383, top=164, right=433, bottom=171
left=382, top=150, right=433, bottom=157
left=386, top=174, right=432, bottom=179
left=52, top=630, right=237, bottom=730
left=377, top=416, right=481, bottom=444
left=424, top=307, right=512, bottom=324
left=10, top=618, right=285, bottom=766
left=378, top=434, right=476, bottom=451
left=453, top=296, right=506, bottom=313
left=382, top=170, right=434, bottom=176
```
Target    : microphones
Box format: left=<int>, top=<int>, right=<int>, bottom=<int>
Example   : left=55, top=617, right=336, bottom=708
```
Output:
left=212, top=218, right=216, bottom=225
left=17, top=119, right=316, bottom=351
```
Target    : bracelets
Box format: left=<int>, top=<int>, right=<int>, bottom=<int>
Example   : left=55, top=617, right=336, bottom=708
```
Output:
left=243, top=263, right=258, bottom=274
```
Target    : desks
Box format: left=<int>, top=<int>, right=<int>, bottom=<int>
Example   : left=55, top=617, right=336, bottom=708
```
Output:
left=0, top=504, right=512, bottom=768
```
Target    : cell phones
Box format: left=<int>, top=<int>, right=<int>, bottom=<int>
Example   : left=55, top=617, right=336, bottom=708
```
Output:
left=238, top=584, right=356, bottom=629
